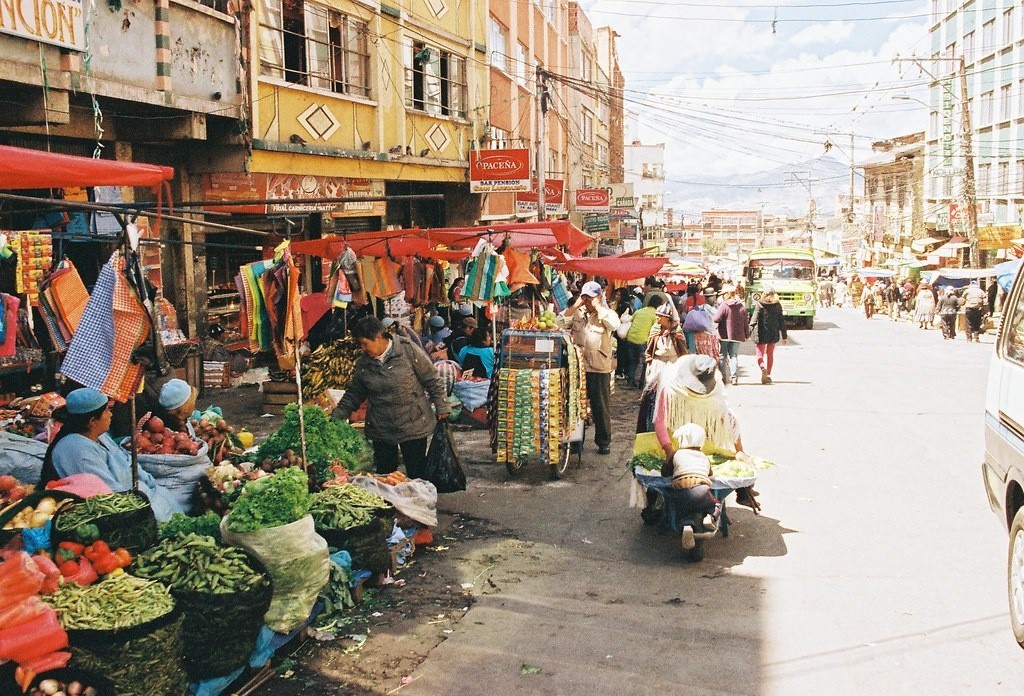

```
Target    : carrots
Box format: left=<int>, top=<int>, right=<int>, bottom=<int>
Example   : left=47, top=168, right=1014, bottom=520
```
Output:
left=0, top=550, right=73, bottom=693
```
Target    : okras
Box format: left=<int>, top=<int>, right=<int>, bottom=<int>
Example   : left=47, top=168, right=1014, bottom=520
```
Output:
left=310, top=498, right=371, bottom=530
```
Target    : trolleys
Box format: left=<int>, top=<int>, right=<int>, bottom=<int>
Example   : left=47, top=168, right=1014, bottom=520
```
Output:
left=497, top=328, right=592, bottom=477
left=635, top=441, right=758, bottom=561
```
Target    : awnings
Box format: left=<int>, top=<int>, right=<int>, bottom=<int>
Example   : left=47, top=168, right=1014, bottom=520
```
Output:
left=815, top=237, right=1022, bottom=298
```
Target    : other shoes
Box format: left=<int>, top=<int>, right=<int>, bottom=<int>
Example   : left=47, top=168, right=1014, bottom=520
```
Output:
left=615, top=372, right=646, bottom=390
left=681, top=526, right=696, bottom=549
left=722, top=373, right=737, bottom=385
left=599, top=443, right=611, bottom=454
left=703, top=515, right=716, bottom=531
left=760, top=367, right=772, bottom=386
left=918, top=323, right=980, bottom=343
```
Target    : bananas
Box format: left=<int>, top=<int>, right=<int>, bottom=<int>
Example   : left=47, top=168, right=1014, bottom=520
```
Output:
left=301, top=329, right=364, bottom=398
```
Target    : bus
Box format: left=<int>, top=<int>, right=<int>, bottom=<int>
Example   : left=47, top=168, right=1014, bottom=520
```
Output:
left=740, top=247, right=822, bottom=329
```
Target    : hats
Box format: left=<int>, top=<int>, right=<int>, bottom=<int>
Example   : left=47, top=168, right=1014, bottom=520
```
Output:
left=429, top=316, right=445, bottom=327
left=702, top=286, right=717, bottom=297
left=158, top=379, right=192, bottom=410
left=66, top=387, right=108, bottom=414
left=581, top=281, right=602, bottom=298
left=673, top=424, right=707, bottom=449
left=655, top=303, right=674, bottom=318
left=459, top=304, right=471, bottom=316
left=461, top=317, right=477, bottom=328
left=674, top=352, right=720, bottom=394
left=382, top=317, right=395, bottom=328
left=718, top=285, right=736, bottom=296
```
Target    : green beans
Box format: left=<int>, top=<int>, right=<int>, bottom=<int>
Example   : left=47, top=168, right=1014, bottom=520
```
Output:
left=55, top=490, right=158, bottom=554
left=41, top=573, right=190, bottom=696
left=133, top=530, right=272, bottom=680
left=312, top=481, right=393, bottom=509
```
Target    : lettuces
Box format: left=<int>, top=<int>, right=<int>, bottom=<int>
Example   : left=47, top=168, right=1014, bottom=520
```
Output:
left=225, top=466, right=330, bottom=635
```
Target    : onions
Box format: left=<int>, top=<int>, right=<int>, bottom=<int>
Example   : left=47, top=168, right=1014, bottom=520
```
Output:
left=121, top=416, right=243, bottom=464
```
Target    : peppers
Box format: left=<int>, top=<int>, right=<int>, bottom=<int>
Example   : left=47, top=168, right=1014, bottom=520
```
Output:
left=51, top=541, right=131, bottom=578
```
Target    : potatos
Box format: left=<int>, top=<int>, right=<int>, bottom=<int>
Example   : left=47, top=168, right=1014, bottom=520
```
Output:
left=1, top=497, right=75, bottom=529
left=0, top=474, right=34, bottom=504
left=32, top=678, right=96, bottom=696
left=261, top=449, right=313, bottom=469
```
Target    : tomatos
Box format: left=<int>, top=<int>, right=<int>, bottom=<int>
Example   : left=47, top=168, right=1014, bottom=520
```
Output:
left=47, top=478, right=69, bottom=489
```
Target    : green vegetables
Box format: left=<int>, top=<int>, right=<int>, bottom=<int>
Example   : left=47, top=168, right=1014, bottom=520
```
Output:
left=232, top=401, right=300, bottom=464
left=300, top=404, right=374, bottom=480
left=156, top=511, right=222, bottom=545
left=617, top=452, right=663, bottom=481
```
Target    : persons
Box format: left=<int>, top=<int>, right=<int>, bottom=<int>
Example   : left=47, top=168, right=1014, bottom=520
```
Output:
left=818, top=265, right=1009, bottom=346
left=40, top=388, right=186, bottom=526
left=157, top=379, right=198, bottom=435
left=329, top=317, right=452, bottom=544
left=343, top=266, right=786, bottom=454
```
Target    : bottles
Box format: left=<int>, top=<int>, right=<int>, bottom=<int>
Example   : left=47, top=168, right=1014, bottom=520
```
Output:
left=237, top=426, right=253, bottom=448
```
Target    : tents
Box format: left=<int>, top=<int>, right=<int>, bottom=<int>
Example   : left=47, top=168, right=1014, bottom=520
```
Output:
left=1, top=146, right=667, bottom=283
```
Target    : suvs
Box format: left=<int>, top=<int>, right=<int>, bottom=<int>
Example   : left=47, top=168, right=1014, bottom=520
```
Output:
left=979, top=256, right=1024, bottom=645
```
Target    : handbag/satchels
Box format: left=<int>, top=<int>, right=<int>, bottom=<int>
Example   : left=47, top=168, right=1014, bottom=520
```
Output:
left=616, top=308, right=631, bottom=339
left=422, top=418, right=467, bottom=494
left=450, top=300, right=460, bottom=311
left=682, top=308, right=711, bottom=332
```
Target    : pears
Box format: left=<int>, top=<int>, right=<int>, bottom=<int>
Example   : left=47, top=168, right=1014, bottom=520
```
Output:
left=538, top=310, right=557, bottom=329
